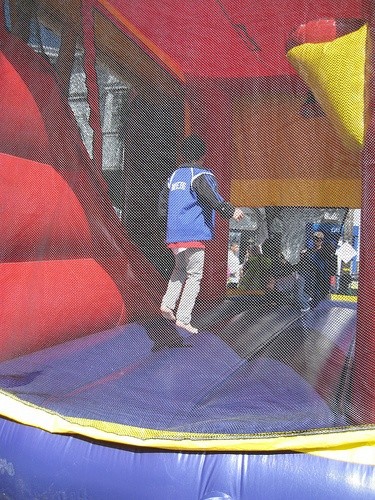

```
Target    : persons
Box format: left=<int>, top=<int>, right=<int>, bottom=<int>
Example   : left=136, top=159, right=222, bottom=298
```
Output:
left=227, top=229, right=332, bottom=312
left=157, top=136, right=244, bottom=335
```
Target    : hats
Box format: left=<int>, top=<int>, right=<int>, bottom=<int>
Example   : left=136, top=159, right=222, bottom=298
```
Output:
left=313, top=232, right=324, bottom=240
